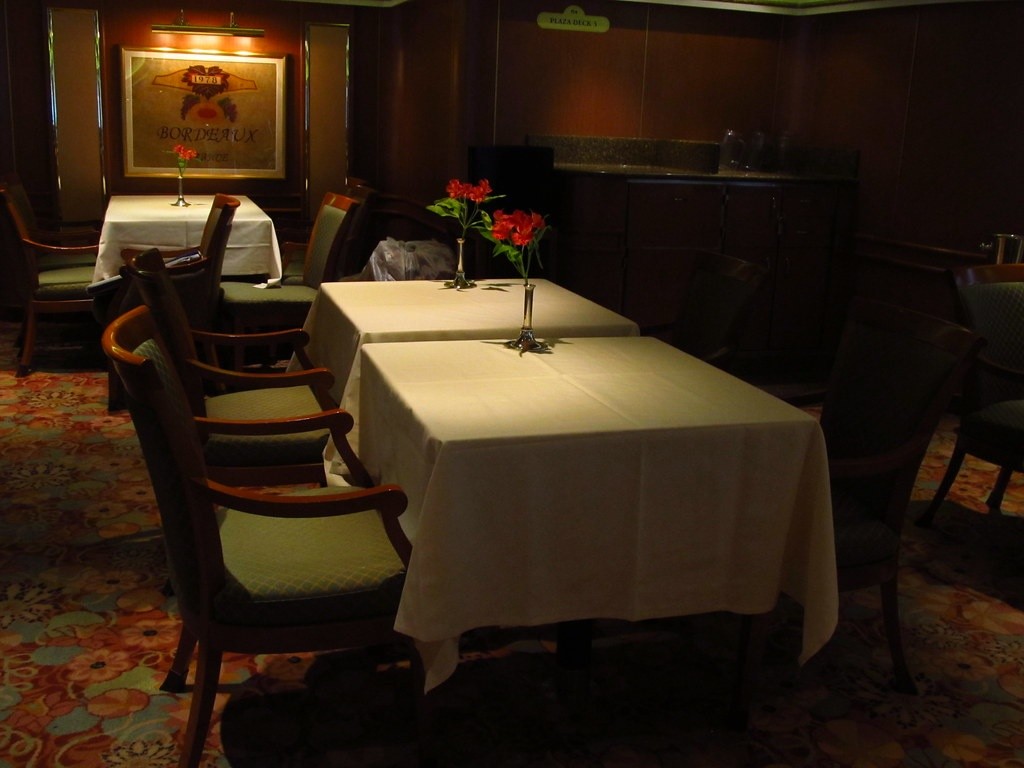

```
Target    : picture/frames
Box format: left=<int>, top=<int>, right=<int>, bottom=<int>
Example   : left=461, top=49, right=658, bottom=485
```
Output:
left=117, top=46, right=291, bottom=183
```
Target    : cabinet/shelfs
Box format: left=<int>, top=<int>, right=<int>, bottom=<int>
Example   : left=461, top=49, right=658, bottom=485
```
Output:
left=525, top=133, right=860, bottom=381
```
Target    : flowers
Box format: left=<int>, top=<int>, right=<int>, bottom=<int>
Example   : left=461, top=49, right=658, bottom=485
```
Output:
left=479, top=209, right=553, bottom=286
left=425, top=177, right=507, bottom=230
left=164, top=144, right=202, bottom=177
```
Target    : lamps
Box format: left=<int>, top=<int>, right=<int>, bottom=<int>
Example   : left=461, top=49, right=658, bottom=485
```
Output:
left=153, top=7, right=265, bottom=39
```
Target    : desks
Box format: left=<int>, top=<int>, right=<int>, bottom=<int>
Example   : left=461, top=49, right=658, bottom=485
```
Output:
left=318, top=277, right=642, bottom=345
left=106, top=195, right=271, bottom=275
left=358, top=336, right=818, bottom=744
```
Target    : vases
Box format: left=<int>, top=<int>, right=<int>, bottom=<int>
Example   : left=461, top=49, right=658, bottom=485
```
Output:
left=170, top=175, right=191, bottom=207
left=443, top=238, right=478, bottom=288
left=506, top=284, right=550, bottom=353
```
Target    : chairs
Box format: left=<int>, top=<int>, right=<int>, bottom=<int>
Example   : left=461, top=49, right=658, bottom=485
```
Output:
left=640, top=247, right=773, bottom=370
left=284, top=179, right=375, bottom=284
left=125, top=247, right=336, bottom=594
left=467, top=144, right=556, bottom=276
left=104, top=303, right=416, bottom=768
left=11, top=172, right=97, bottom=265
left=216, top=192, right=359, bottom=381
left=749, top=297, right=988, bottom=695
left=913, top=262, right=1024, bottom=532
left=1, top=182, right=101, bottom=374
left=84, top=190, right=242, bottom=413
